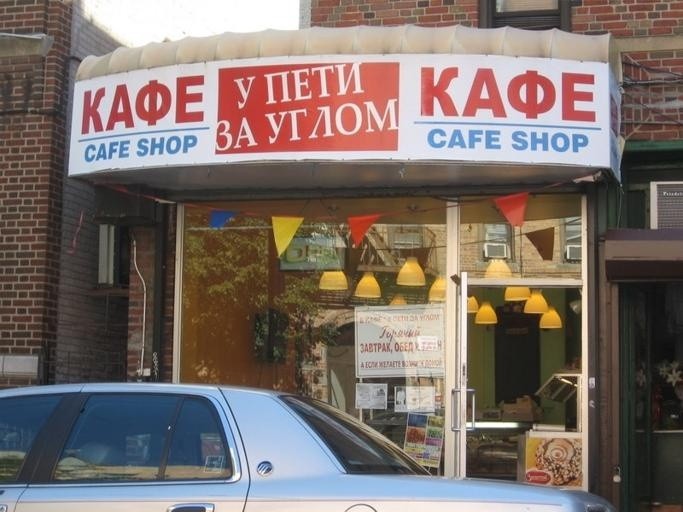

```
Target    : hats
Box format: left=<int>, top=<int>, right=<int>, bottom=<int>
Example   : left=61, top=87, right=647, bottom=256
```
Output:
left=0, top=381, right=616, bottom=512
left=368, top=410, right=531, bottom=477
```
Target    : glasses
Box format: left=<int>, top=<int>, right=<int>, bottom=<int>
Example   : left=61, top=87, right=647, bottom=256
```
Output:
left=318, top=203, right=563, bottom=331
